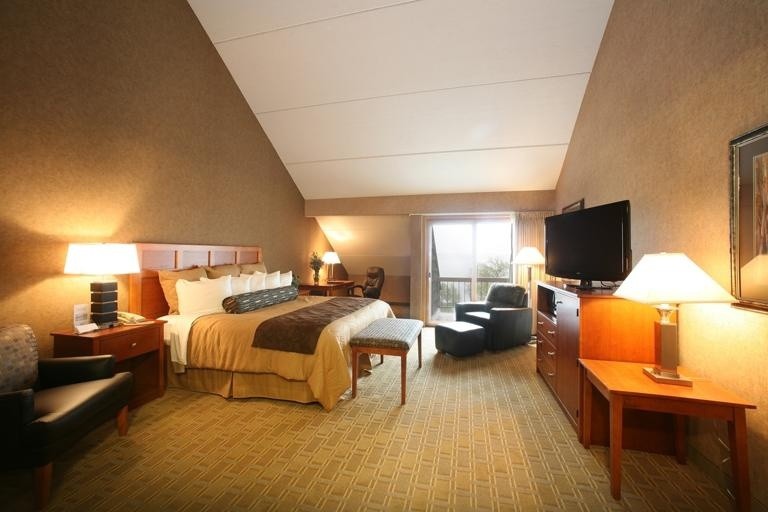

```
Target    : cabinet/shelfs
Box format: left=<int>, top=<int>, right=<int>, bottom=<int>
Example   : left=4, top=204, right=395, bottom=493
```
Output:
left=536, top=279, right=679, bottom=455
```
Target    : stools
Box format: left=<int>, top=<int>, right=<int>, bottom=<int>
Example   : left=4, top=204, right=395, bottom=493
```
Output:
left=435, top=321, right=485, bottom=357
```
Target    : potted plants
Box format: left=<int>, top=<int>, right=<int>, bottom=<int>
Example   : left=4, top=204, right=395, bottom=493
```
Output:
left=308, top=251, right=324, bottom=282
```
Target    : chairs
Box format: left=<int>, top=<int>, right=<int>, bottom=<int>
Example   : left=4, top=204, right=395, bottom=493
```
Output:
left=455, top=282, right=533, bottom=351
left=347, top=266, right=384, bottom=298
left=0, top=324, right=134, bottom=512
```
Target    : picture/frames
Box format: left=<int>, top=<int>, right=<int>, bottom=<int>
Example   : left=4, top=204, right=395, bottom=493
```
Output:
left=561, top=199, right=583, bottom=214
left=728, top=126, right=768, bottom=316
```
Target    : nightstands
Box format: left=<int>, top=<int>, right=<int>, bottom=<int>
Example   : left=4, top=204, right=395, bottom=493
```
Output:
left=50, top=320, right=168, bottom=411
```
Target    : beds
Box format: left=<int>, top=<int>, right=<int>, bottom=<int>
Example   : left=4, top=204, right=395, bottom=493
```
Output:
left=129, top=243, right=396, bottom=412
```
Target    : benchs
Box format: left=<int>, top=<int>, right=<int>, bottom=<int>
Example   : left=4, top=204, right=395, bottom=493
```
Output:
left=350, top=317, right=424, bottom=404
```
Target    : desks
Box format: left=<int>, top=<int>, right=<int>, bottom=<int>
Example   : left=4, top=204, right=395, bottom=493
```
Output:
left=578, top=357, right=758, bottom=511
left=297, top=280, right=357, bottom=296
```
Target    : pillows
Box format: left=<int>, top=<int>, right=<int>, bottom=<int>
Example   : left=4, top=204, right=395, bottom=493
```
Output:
left=157, top=266, right=208, bottom=315
left=242, top=272, right=266, bottom=292
left=264, top=270, right=280, bottom=290
left=232, top=276, right=251, bottom=295
left=205, top=264, right=240, bottom=279
left=241, top=262, right=267, bottom=276
left=177, top=275, right=232, bottom=315
left=281, top=270, right=292, bottom=288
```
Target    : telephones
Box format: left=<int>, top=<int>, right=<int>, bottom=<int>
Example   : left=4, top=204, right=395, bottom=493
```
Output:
left=117, top=312, right=146, bottom=324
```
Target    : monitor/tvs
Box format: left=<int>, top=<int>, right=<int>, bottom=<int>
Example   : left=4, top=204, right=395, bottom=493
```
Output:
left=545, top=199, right=633, bottom=290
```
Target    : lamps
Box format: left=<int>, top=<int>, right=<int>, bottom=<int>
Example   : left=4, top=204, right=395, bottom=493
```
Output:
left=65, top=243, right=141, bottom=324
left=511, top=247, right=545, bottom=308
left=611, top=252, right=742, bottom=386
left=321, top=252, right=341, bottom=280
